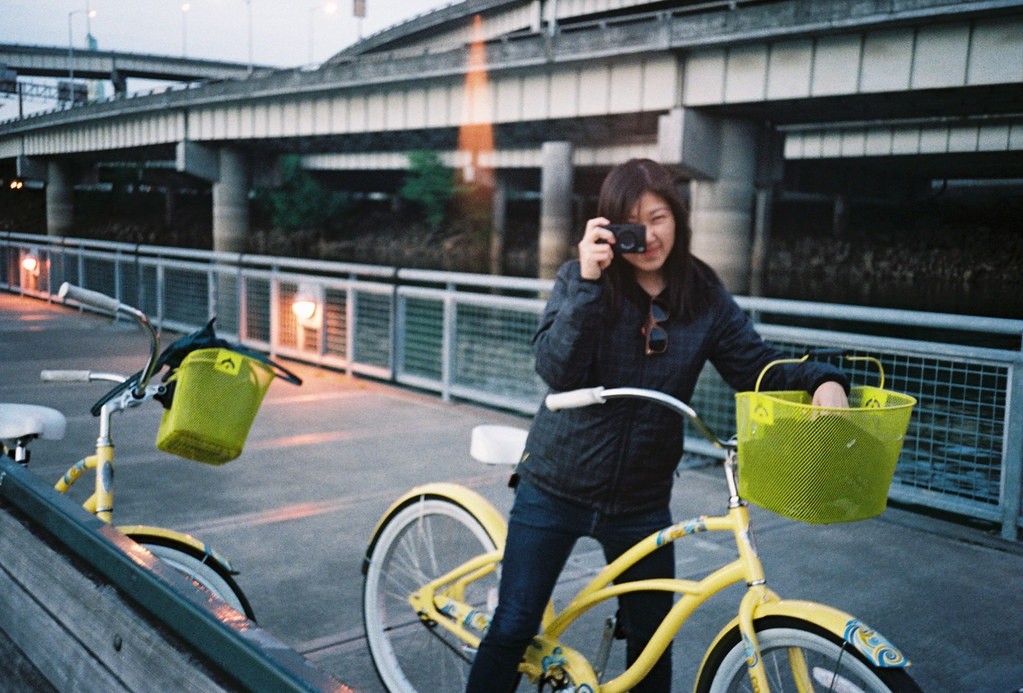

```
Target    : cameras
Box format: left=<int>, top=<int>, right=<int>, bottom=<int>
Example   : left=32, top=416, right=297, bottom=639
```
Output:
left=594, top=224, right=647, bottom=254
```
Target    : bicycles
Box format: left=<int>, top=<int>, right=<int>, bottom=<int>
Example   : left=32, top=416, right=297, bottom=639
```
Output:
left=356, top=348, right=922, bottom=692
left=0, top=280, right=276, bottom=622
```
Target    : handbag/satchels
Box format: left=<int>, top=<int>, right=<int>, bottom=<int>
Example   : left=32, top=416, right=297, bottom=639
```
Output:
left=153, top=328, right=302, bottom=409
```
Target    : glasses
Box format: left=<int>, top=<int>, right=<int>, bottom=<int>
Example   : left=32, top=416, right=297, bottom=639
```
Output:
left=641, top=296, right=672, bottom=356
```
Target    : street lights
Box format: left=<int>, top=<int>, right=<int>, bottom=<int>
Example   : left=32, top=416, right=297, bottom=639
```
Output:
left=66, top=8, right=97, bottom=106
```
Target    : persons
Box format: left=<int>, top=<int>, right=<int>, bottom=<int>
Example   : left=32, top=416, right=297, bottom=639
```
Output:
left=467, top=159, right=851, bottom=693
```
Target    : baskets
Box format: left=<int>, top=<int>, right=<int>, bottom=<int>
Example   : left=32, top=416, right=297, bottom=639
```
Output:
left=733, top=382, right=919, bottom=524
left=154, top=348, right=276, bottom=466
